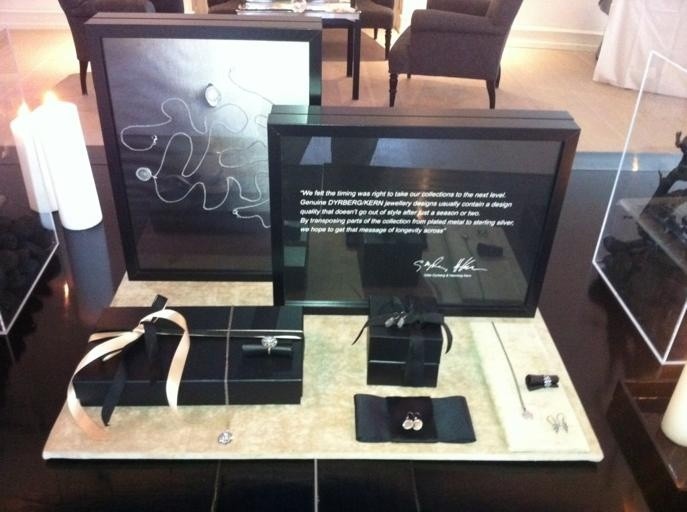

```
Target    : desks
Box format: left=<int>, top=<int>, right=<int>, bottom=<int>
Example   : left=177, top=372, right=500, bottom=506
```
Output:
left=0, top=146, right=687, bottom=512
left=318, top=11, right=362, bottom=101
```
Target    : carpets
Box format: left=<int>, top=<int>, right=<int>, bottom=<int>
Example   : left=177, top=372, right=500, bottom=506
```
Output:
left=43, top=271, right=605, bottom=512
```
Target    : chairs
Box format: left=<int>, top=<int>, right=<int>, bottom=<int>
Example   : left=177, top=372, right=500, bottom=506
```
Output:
left=209, top=1, right=396, bottom=36
left=59, top=0, right=188, bottom=95
left=386, top=1, right=523, bottom=109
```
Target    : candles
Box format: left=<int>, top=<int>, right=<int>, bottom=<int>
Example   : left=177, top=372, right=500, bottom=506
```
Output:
left=9, top=101, right=58, bottom=214
left=31, top=90, right=104, bottom=231
left=660, top=360, right=686, bottom=447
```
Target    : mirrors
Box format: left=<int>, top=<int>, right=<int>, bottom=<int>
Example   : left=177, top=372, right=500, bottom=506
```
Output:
left=0, top=17, right=62, bottom=336
